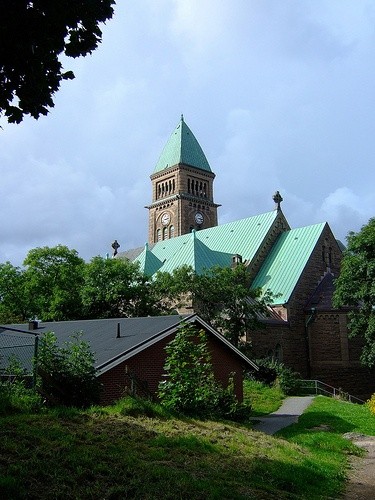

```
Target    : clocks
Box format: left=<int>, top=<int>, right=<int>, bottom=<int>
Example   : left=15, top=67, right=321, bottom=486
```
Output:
left=161, top=213, right=170, bottom=226
left=194, top=212, right=204, bottom=224
